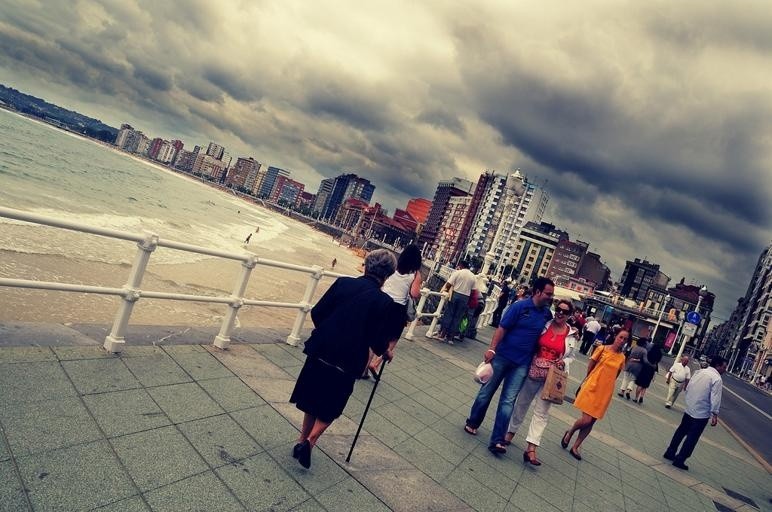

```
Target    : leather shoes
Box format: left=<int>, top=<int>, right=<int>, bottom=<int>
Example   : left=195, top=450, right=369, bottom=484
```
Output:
left=561, top=431, right=571, bottom=448
left=570, top=447, right=582, bottom=460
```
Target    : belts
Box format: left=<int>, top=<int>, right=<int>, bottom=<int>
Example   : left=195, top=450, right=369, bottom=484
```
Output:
left=672, top=377, right=683, bottom=383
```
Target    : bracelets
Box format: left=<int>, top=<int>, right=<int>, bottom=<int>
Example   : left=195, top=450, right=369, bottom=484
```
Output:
left=488, top=349, right=496, bottom=355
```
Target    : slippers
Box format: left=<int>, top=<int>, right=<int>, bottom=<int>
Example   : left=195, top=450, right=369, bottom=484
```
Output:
left=464, top=424, right=476, bottom=435
left=368, top=366, right=381, bottom=381
left=361, top=375, right=371, bottom=379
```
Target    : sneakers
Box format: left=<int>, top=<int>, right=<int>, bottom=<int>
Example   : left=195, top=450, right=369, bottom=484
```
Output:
left=293, top=443, right=301, bottom=458
left=297, top=440, right=311, bottom=468
left=616, top=392, right=688, bottom=470
left=488, top=443, right=506, bottom=453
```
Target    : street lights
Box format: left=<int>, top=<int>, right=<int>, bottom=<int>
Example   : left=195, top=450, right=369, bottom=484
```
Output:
left=650, top=293, right=673, bottom=343
left=665, top=282, right=707, bottom=384
left=474, top=166, right=530, bottom=293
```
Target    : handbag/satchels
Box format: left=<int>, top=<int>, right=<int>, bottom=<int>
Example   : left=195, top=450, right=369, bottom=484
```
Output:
left=541, top=367, right=568, bottom=405
left=406, top=299, right=416, bottom=322
left=529, top=358, right=566, bottom=380
left=459, top=318, right=468, bottom=333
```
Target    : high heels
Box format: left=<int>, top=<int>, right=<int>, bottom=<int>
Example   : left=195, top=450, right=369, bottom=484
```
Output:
left=504, top=440, right=511, bottom=445
left=523, top=451, right=541, bottom=466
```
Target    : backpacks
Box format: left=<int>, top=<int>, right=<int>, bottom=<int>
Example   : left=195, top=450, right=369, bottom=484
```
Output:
left=647, top=343, right=662, bottom=364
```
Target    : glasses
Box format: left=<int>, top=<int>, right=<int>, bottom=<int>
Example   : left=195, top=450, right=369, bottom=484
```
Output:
left=555, top=307, right=570, bottom=315
left=544, top=292, right=555, bottom=298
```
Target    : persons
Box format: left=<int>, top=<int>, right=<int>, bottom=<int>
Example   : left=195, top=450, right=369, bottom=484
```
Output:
left=465, top=277, right=580, bottom=454
left=733, top=364, right=772, bottom=393
left=245, top=234, right=252, bottom=243
left=255, top=226, right=259, bottom=233
left=664, top=355, right=727, bottom=470
left=332, top=258, right=337, bottom=267
left=358, top=263, right=364, bottom=273
left=551, top=295, right=658, bottom=355
left=561, top=327, right=633, bottom=460
left=665, top=354, right=690, bottom=408
left=292, top=249, right=398, bottom=469
left=423, top=244, right=531, bottom=344
left=618, top=338, right=657, bottom=399
left=504, top=299, right=579, bottom=465
left=630, top=343, right=662, bottom=403
left=360, top=244, right=421, bottom=381
